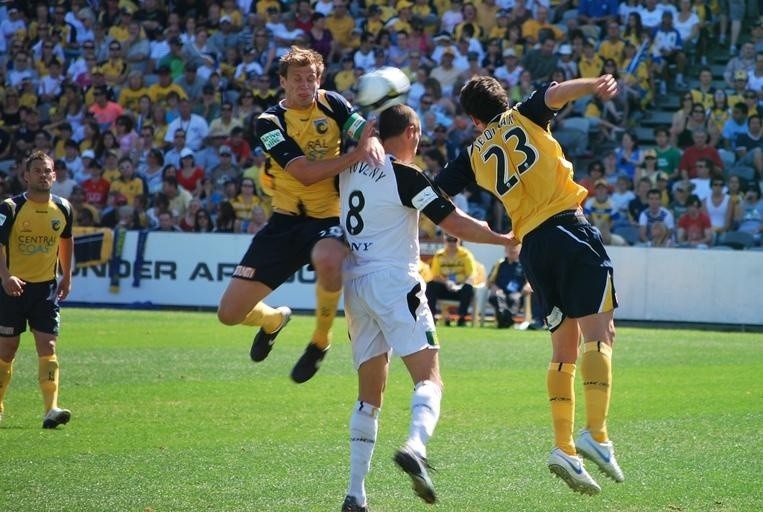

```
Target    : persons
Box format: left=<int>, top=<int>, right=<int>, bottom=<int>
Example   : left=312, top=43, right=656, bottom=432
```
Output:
left=571, top=0, right=762, bottom=250
left=76, top=150, right=218, bottom=232
left=434, top=74, right=624, bottom=495
left=464, top=1, right=571, bottom=75
left=427, top=230, right=476, bottom=325
left=0, top=0, right=263, bottom=147
left=487, top=245, right=542, bottom=329
left=0, top=152, right=75, bottom=427
left=264, top=3, right=316, bottom=53
left=335, top=104, right=519, bottom=511
left=218, top=52, right=385, bottom=384
left=315, top=1, right=463, bottom=104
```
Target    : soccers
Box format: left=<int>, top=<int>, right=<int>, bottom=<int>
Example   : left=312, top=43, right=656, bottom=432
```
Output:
left=361, top=66, right=410, bottom=114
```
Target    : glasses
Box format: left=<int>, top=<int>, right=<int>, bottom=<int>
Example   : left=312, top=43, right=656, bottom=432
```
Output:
left=259, top=79, right=271, bottom=84
left=241, top=184, right=254, bottom=188
left=488, top=43, right=498, bottom=47
left=683, top=97, right=691, bottom=101
left=695, top=165, right=707, bottom=169
left=54, top=11, right=65, bottom=15
left=421, top=100, right=433, bottom=105
left=221, top=108, right=232, bottom=112
left=83, top=46, right=95, bottom=50
left=93, top=91, right=105, bottom=96
left=116, top=119, right=127, bottom=125
left=141, top=134, right=152, bottom=138
left=13, top=45, right=24, bottom=50
left=367, top=40, right=375, bottom=44
left=419, top=143, right=431, bottom=147
left=216, top=208, right=221, bottom=212
left=694, top=109, right=705, bottom=113
left=743, top=95, right=757, bottom=99
left=16, top=59, right=27, bottom=63
left=6, top=94, right=16, bottom=98
left=255, top=34, right=266, bottom=38
left=44, top=45, right=54, bottom=49
left=434, top=129, right=446, bottom=133
left=445, top=238, right=458, bottom=242
left=175, top=135, right=185, bottom=139
left=185, top=68, right=197, bottom=73
left=591, top=168, right=603, bottom=172
left=467, top=58, right=477, bottom=61
left=197, top=216, right=208, bottom=220
left=677, top=188, right=684, bottom=192
left=712, top=183, right=725, bottom=187
left=109, top=47, right=121, bottom=51
left=38, top=26, right=48, bottom=31
left=241, top=95, right=253, bottom=99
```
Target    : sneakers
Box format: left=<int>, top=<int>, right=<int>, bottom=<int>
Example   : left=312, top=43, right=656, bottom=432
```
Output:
left=43, top=408, right=70, bottom=429
left=250, top=306, right=292, bottom=362
left=393, top=445, right=437, bottom=504
left=575, top=430, right=624, bottom=482
left=291, top=342, right=330, bottom=383
left=342, top=496, right=368, bottom=512
left=529, top=320, right=544, bottom=329
left=458, top=318, right=466, bottom=326
left=549, top=447, right=601, bottom=496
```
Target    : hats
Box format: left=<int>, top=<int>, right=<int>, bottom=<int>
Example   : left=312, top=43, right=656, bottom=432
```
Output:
left=432, top=31, right=451, bottom=41
left=443, top=232, right=460, bottom=240
left=89, top=159, right=104, bottom=168
left=81, top=149, right=94, bottom=159
left=241, top=46, right=257, bottom=56
left=657, top=172, right=669, bottom=181
left=220, top=16, right=233, bottom=24
left=558, top=45, right=573, bottom=55
left=644, top=149, right=657, bottom=159
left=180, top=147, right=194, bottom=158
left=27, top=107, right=40, bottom=116
left=584, top=36, right=595, bottom=47
left=91, top=66, right=104, bottom=77
left=594, top=179, right=608, bottom=189
left=168, top=36, right=181, bottom=44
left=442, top=47, right=455, bottom=58
left=734, top=69, right=749, bottom=82
left=396, top=0, right=414, bottom=12
left=120, top=5, right=133, bottom=16
left=218, top=145, right=231, bottom=156
left=154, top=64, right=171, bottom=75
left=496, top=8, right=511, bottom=19
left=420, top=135, right=432, bottom=143
left=105, top=149, right=117, bottom=158
left=503, top=48, right=516, bottom=58
left=433, top=123, right=448, bottom=130
left=467, top=52, right=478, bottom=59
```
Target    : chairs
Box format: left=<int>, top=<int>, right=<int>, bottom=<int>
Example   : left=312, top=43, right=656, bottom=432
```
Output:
left=718, top=230, right=756, bottom=247
left=745, top=245, right=763, bottom=252
left=433, top=262, right=481, bottom=327
left=728, top=163, right=755, bottom=179
left=711, top=244, right=737, bottom=251
